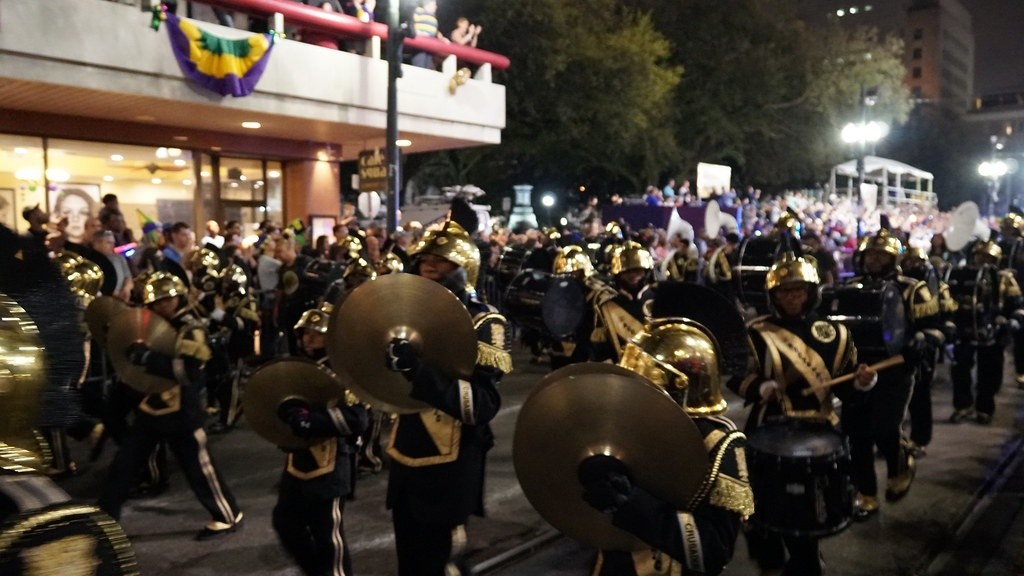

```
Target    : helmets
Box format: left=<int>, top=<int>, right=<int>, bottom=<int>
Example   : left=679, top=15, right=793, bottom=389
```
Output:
left=1001, top=213, right=1024, bottom=238
left=57, top=254, right=104, bottom=310
left=141, top=272, right=189, bottom=305
left=615, top=318, right=728, bottom=416
left=610, top=247, right=656, bottom=277
left=294, top=309, right=331, bottom=333
left=405, top=231, right=482, bottom=288
left=550, top=247, right=597, bottom=280
left=764, top=250, right=821, bottom=295
left=972, top=240, right=1003, bottom=262
left=853, top=228, right=905, bottom=265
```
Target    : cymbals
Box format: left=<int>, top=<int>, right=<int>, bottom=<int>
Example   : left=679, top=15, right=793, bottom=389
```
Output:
left=511, top=362, right=710, bottom=552
left=326, top=271, right=479, bottom=416
left=85, top=296, right=184, bottom=395
left=242, top=356, right=346, bottom=452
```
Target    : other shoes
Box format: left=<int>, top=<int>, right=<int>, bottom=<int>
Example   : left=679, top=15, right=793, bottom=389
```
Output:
left=854, top=497, right=882, bottom=520
left=977, top=411, right=996, bottom=425
left=948, top=407, right=974, bottom=424
left=203, top=512, right=244, bottom=535
left=883, top=459, right=917, bottom=503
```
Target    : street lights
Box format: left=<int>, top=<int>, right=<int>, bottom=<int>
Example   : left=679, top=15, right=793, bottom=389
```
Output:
left=980, top=138, right=1008, bottom=225
left=839, top=80, right=889, bottom=246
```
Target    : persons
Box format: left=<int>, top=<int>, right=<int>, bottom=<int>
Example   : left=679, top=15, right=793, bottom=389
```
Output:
left=726, top=248, right=879, bottom=576
left=0, top=293, right=142, bottom=576
left=512, top=316, right=753, bottom=576
left=330, top=228, right=512, bottom=575
left=946, top=212, right=1024, bottom=424
left=84, top=272, right=246, bottom=535
left=452, top=17, right=484, bottom=79
left=644, top=177, right=951, bottom=262
left=249, top=300, right=369, bottom=576
left=0, top=190, right=730, bottom=354
left=814, top=229, right=944, bottom=521
left=408, top=0, right=450, bottom=68
left=310, top=1, right=378, bottom=56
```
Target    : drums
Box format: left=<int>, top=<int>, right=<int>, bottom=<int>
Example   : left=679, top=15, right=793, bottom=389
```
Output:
left=940, top=263, right=1001, bottom=347
left=815, top=278, right=910, bottom=362
left=732, top=231, right=788, bottom=307
left=496, top=245, right=546, bottom=293
left=745, top=418, right=854, bottom=538
left=503, top=269, right=586, bottom=340
